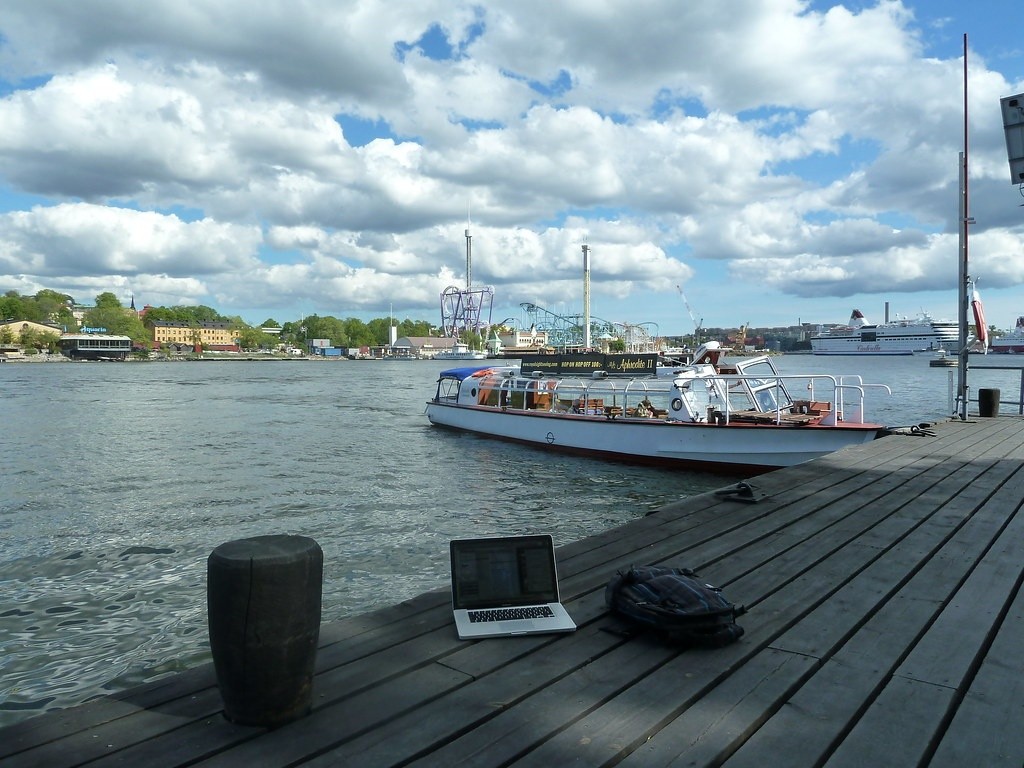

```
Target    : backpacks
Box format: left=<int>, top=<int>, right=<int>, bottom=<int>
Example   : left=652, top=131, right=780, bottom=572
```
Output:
left=605, top=566, right=745, bottom=649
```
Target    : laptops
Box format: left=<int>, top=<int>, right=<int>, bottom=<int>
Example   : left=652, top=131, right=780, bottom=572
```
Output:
left=450, top=534, right=577, bottom=639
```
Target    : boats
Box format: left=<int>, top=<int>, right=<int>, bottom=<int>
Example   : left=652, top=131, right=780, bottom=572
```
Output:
left=930, top=353, right=959, bottom=368
left=990, top=316, right=1024, bottom=355
left=433, top=337, right=488, bottom=361
left=809, top=304, right=994, bottom=356
left=383, top=349, right=417, bottom=360
left=425, top=341, right=892, bottom=479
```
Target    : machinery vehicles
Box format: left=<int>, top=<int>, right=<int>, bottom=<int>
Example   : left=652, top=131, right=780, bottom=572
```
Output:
left=732, top=320, right=750, bottom=351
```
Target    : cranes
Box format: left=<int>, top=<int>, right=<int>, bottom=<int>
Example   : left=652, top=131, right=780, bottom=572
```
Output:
left=676, top=283, right=704, bottom=334
